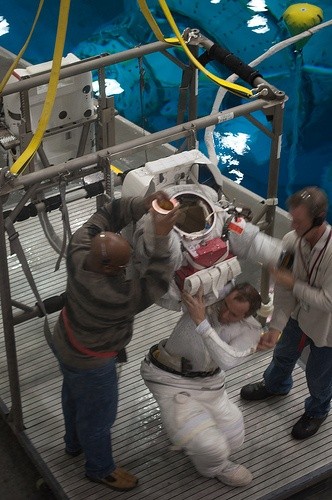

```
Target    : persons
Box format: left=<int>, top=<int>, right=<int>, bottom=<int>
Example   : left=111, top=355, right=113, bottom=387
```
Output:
left=138, top=282, right=264, bottom=488
left=240, top=186, right=331, bottom=441
left=50, top=190, right=184, bottom=491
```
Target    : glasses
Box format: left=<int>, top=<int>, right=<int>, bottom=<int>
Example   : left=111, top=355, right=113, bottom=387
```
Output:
left=101, top=264, right=129, bottom=272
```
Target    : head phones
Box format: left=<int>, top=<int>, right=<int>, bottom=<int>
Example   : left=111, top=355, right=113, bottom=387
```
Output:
left=301, top=216, right=325, bottom=239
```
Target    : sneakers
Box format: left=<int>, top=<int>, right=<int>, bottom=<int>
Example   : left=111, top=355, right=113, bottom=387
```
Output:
left=85, top=467, right=139, bottom=492
left=292, top=413, right=328, bottom=439
left=216, top=459, right=253, bottom=487
left=241, top=380, right=289, bottom=400
